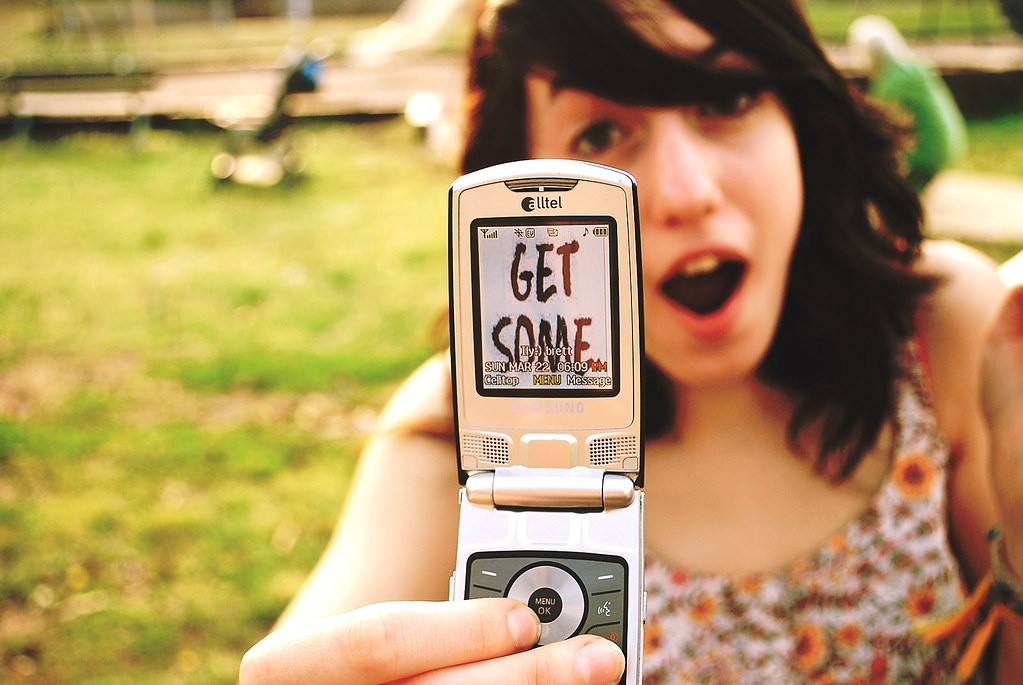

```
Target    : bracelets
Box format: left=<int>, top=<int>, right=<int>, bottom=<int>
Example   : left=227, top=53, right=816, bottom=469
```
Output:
left=985, top=530, right=1022, bottom=614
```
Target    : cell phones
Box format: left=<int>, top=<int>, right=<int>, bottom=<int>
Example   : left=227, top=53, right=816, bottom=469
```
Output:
left=445, top=157, right=647, bottom=685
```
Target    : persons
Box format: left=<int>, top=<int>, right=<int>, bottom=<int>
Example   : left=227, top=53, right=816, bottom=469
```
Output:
left=235, top=1, right=1023, bottom=684
left=847, top=17, right=968, bottom=192
